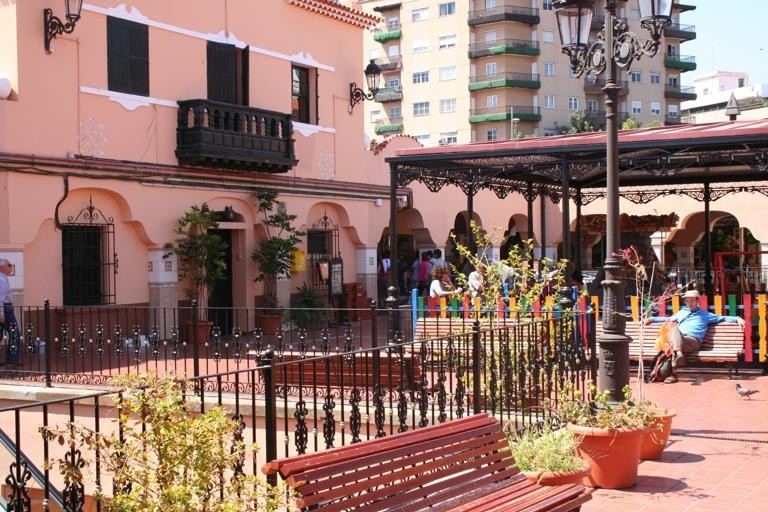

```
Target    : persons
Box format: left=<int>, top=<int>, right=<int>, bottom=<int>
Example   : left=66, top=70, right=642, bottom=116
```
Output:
left=380, top=251, right=391, bottom=308
left=0, top=260, right=24, bottom=367
left=416, top=253, right=429, bottom=293
left=468, top=264, right=489, bottom=305
left=410, top=251, right=420, bottom=290
left=425, top=251, right=435, bottom=286
left=430, top=266, right=463, bottom=298
left=644, top=290, right=746, bottom=383
left=401, top=268, right=413, bottom=294
left=432, top=249, right=443, bottom=267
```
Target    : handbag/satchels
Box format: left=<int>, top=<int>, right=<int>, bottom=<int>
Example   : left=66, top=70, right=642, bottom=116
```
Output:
left=655, top=319, right=674, bottom=349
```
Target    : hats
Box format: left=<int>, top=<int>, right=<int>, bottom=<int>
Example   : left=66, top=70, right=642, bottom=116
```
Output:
left=682, top=290, right=700, bottom=301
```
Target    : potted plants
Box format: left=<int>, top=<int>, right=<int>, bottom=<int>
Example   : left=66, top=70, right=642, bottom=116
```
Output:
left=250, top=187, right=307, bottom=334
left=162, top=202, right=229, bottom=344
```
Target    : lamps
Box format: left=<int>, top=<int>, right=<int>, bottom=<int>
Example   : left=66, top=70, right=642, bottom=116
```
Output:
left=348, top=58, right=381, bottom=113
left=44, top=0, right=83, bottom=55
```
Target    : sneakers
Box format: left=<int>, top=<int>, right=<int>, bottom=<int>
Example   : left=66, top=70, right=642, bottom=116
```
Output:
left=665, top=375, right=678, bottom=383
left=677, top=354, right=686, bottom=367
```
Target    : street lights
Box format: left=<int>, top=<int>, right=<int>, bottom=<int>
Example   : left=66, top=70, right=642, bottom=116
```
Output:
left=554, top=1, right=674, bottom=417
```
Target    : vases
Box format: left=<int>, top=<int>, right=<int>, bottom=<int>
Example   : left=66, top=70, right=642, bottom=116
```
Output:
left=638, top=400, right=678, bottom=459
left=499, top=412, right=592, bottom=512
left=541, top=379, right=657, bottom=488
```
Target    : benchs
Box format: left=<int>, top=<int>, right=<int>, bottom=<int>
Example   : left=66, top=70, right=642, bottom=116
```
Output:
left=258, top=356, right=439, bottom=392
left=403, top=318, right=745, bottom=379
left=262, top=412, right=592, bottom=512
